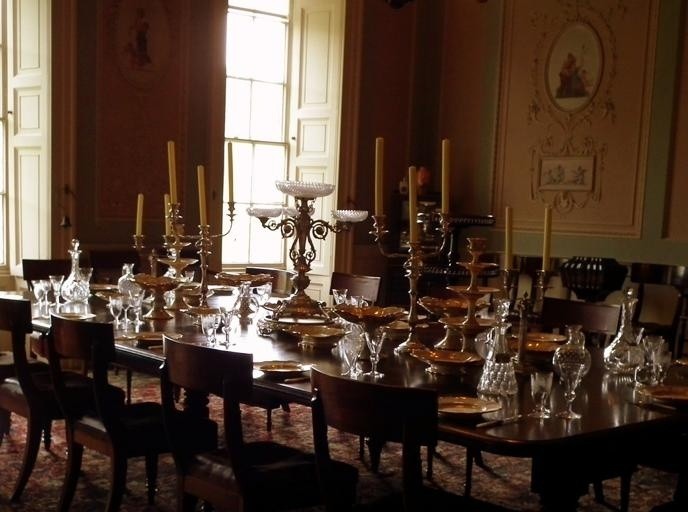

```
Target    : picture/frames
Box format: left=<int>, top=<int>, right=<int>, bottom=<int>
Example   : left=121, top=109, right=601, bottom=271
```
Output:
left=488, top=2, right=663, bottom=238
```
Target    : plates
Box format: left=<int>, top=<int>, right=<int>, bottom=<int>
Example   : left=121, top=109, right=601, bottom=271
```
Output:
left=53, top=283, right=688, bottom=426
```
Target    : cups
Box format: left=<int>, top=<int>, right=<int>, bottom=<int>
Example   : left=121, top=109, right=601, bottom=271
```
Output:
left=184, top=271, right=195, bottom=282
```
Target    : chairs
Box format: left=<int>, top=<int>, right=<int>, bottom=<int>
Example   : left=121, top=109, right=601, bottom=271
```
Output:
left=1, top=298, right=127, bottom=512
left=156, top=257, right=688, bottom=393
left=158, top=334, right=359, bottom=511
left=309, top=367, right=453, bottom=512
left=47, top=314, right=218, bottom=512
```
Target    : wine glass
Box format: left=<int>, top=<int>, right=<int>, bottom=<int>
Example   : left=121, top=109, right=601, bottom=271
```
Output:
left=28, top=275, right=66, bottom=320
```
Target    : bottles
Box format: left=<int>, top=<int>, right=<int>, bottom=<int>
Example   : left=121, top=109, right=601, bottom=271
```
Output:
left=162, top=246, right=187, bottom=285
left=57, top=237, right=92, bottom=304
left=117, top=263, right=145, bottom=294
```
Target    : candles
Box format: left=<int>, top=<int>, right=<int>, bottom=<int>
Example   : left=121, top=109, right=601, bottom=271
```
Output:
left=165, top=193, right=173, bottom=236
left=375, top=137, right=386, bottom=218
left=441, top=139, right=450, bottom=214
left=227, top=142, right=234, bottom=202
left=168, top=141, right=179, bottom=203
left=503, top=205, right=513, bottom=270
left=541, top=207, right=552, bottom=270
left=197, top=166, right=208, bottom=225
left=408, top=167, right=417, bottom=243
left=134, top=194, right=145, bottom=236
